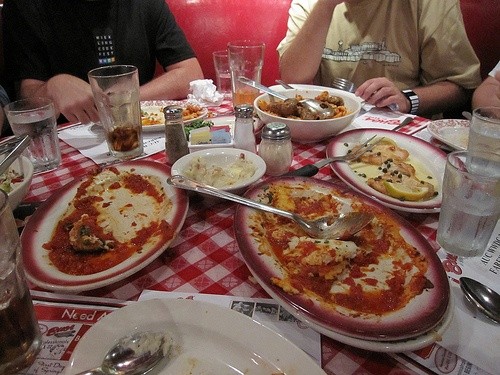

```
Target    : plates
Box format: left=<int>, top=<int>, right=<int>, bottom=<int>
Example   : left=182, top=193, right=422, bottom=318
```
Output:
left=428, top=119, right=469, bottom=151
left=21, top=84, right=454, bottom=375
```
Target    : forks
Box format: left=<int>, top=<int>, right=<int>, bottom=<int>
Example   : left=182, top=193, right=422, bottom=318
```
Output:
left=332, top=77, right=398, bottom=111
left=285, top=134, right=385, bottom=177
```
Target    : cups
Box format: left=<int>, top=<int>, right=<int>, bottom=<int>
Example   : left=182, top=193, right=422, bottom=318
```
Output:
left=466, top=106, right=500, bottom=175
left=88, top=64, right=143, bottom=161
left=437, top=150, right=500, bottom=257
left=4, top=96, right=61, bottom=171
left=213, top=40, right=265, bottom=105
left=0, top=189, right=42, bottom=375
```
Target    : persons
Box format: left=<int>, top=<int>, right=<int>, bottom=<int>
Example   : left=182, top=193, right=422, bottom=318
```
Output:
left=0, top=84, right=10, bottom=138
left=472, top=61, right=500, bottom=121
left=276, top=0, right=482, bottom=115
left=0, top=0, right=203, bottom=125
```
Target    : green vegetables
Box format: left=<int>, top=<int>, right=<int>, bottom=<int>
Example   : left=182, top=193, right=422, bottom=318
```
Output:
left=183, top=118, right=215, bottom=141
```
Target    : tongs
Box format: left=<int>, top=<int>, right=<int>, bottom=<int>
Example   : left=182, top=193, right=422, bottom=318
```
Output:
left=0, top=132, right=33, bottom=174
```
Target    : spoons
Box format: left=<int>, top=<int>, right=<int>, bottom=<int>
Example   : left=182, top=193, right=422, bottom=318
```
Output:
left=75, top=331, right=174, bottom=374
left=238, top=76, right=334, bottom=118
left=167, top=175, right=374, bottom=239
left=459, top=276, right=500, bottom=324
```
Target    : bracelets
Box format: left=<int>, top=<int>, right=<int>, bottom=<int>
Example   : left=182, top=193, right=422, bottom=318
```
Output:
left=401, top=89, right=420, bottom=114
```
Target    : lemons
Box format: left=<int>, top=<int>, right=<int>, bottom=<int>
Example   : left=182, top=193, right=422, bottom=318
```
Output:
left=382, top=181, right=428, bottom=200
left=367, top=136, right=397, bottom=147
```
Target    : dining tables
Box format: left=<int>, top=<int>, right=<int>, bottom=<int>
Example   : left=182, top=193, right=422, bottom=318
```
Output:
left=0, top=94, right=452, bottom=375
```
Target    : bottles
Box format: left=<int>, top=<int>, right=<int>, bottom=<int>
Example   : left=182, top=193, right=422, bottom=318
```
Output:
left=233, top=103, right=259, bottom=157
left=259, top=121, right=294, bottom=176
left=162, top=106, right=190, bottom=165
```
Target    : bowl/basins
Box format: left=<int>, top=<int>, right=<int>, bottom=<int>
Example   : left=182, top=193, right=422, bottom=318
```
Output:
left=0, top=154, right=34, bottom=210
left=253, top=87, right=361, bottom=143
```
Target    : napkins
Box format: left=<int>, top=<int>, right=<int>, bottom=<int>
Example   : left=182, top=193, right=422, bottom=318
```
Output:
left=187, top=79, right=224, bottom=108
